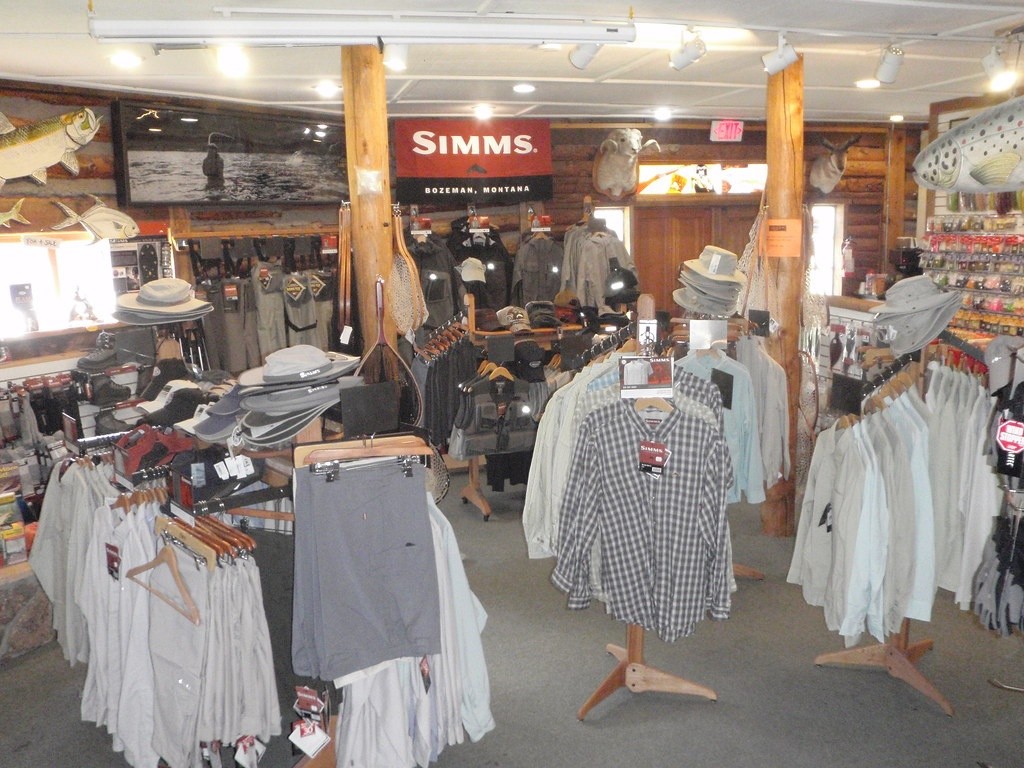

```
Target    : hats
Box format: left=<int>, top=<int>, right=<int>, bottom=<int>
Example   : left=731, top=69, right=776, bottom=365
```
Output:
left=598, top=266, right=639, bottom=297
left=111, top=277, right=215, bottom=328
left=597, top=304, right=625, bottom=317
left=524, top=301, right=562, bottom=329
left=554, top=288, right=582, bottom=310
left=458, top=256, right=486, bottom=284
left=136, top=341, right=365, bottom=448
left=869, top=273, right=962, bottom=357
left=515, top=339, right=545, bottom=384
left=475, top=305, right=505, bottom=334
left=496, top=306, right=533, bottom=335
left=669, top=245, right=748, bottom=318
left=982, top=333, right=1023, bottom=398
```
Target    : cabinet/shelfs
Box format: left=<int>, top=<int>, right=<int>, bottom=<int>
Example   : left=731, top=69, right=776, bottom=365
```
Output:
left=0, top=206, right=1024, bottom=768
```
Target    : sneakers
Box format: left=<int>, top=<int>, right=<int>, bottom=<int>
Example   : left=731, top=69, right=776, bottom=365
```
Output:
left=90, top=375, right=131, bottom=406
left=107, top=423, right=198, bottom=489
left=76, top=331, right=124, bottom=373
left=91, top=408, right=135, bottom=437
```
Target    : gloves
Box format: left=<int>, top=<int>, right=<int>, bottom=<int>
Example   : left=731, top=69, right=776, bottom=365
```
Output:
left=974, top=514, right=1023, bottom=636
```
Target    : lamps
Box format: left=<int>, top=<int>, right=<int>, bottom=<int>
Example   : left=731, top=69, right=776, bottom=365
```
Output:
left=980, top=46, right=1018, bottom=93
left=570, top=42, right=607, bottom=69
left=761, top=33, right=800, bottom=75
left=669, top=37, right=707, bottom=72
left=876, top=47, right=904, bottom=83
left=87, top=18, right=638, bottom=46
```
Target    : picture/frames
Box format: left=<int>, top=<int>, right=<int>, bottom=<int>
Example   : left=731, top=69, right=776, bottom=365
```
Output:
left=111, top=98, right=350, bottom=206
